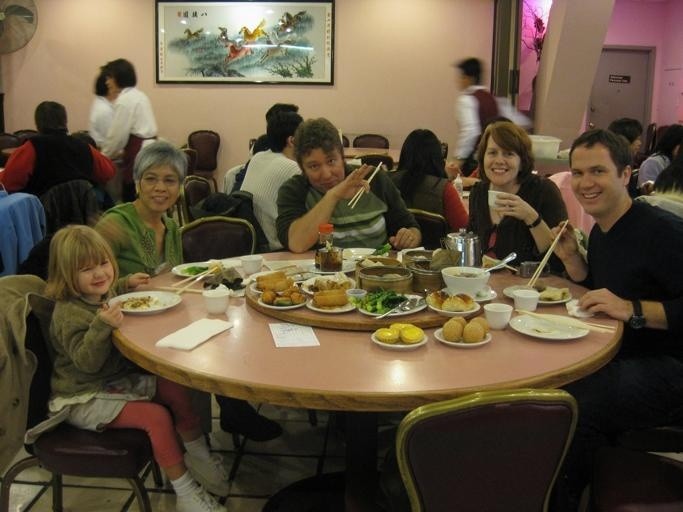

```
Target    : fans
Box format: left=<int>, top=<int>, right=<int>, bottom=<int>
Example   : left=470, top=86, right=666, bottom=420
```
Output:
left=0, top=1, right=37, bottom=54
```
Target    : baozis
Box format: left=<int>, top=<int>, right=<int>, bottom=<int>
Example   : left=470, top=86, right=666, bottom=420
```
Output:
left=426, top=290, right=475, bottom=313
left=362, top=259, right=373, bottom=266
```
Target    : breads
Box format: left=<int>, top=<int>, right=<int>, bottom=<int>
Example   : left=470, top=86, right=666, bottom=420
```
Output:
left=373, top=322, right=423, bottom=344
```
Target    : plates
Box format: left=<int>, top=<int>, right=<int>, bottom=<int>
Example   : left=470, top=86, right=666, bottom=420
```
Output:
left=110, top=291, right=181, bottom=314
left=510, top=313, right=590, bottom=340
left=434, top=328, right=491, bottom=347
left=249, top=262, right=499, bottom=318
left=173, top=262, right=214, bottom=278
left=371, top=331, right=428, bottom=349
left=342, top=248, right=381, bottom=260
left=503, top=285, right=573, bottom=305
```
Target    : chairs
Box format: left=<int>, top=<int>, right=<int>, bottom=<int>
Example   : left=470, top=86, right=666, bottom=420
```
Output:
left=41, top=178, right=97, bottom=234
left=0, top=129, right=38, bottom=167
left=405, top=208, right=448, bottom=249
left=340, top=133, right=389, bottom=149
left=643, top=451, right=683, bottom=471
left=395, top=388, right=580, bottom=511
left=0, top=275, right=164, bottom=511
left=17, top=234, right=49, bottom=281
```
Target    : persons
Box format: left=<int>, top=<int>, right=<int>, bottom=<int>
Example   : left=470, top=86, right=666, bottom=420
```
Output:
left=98, top=58, right=157, bottom=204
left=43, top=101, right=469, bottom=510
left=463, top=117, right=683, bottom=510
left=0, top=100, right=115, bottom=198
left=86, top=65, right=124, bottom=163
left=444, top=55, right=534, bottom=190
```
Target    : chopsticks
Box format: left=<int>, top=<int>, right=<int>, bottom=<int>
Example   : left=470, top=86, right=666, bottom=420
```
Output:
left=347, top=162, right=383, bottom=211
left=521, top=310, right=616, bottom=335
left=172, top=265, right=217, bottom=296
left=526, top=219, right=568, bottom=287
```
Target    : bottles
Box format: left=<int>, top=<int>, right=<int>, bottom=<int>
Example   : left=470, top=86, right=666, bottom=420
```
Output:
left=314, top=223, right=335, bottom=268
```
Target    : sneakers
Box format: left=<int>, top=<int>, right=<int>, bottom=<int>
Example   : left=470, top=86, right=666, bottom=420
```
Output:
left=176, top=489, right=229, bottom=511
left=220, top=408, right=284, bottom=441
left=183, top=450, right=235, bottom=497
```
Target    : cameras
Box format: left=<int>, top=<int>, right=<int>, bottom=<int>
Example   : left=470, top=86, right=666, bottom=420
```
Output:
left=521, top=261, right=550, bottom=279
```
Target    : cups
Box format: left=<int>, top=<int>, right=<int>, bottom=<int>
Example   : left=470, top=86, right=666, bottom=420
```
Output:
left=202, top=290, right=230, bottom=315
left=486, top=189, right=512, bottom=214
left=513, top=290, right=539, bottom=312
left=240, top=255, right=263, bottom=275
left=483, top=303, right=513, bottom=331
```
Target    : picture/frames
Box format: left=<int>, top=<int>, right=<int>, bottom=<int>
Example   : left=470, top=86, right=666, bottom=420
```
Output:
left=155, top=0, right=335, bottom=86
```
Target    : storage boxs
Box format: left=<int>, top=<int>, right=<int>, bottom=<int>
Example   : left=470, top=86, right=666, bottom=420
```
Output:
left=530, top=135, right=562, bottom=159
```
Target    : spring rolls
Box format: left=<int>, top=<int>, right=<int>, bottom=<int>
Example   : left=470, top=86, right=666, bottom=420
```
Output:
left=256, top=272, right=347, bottom=305
